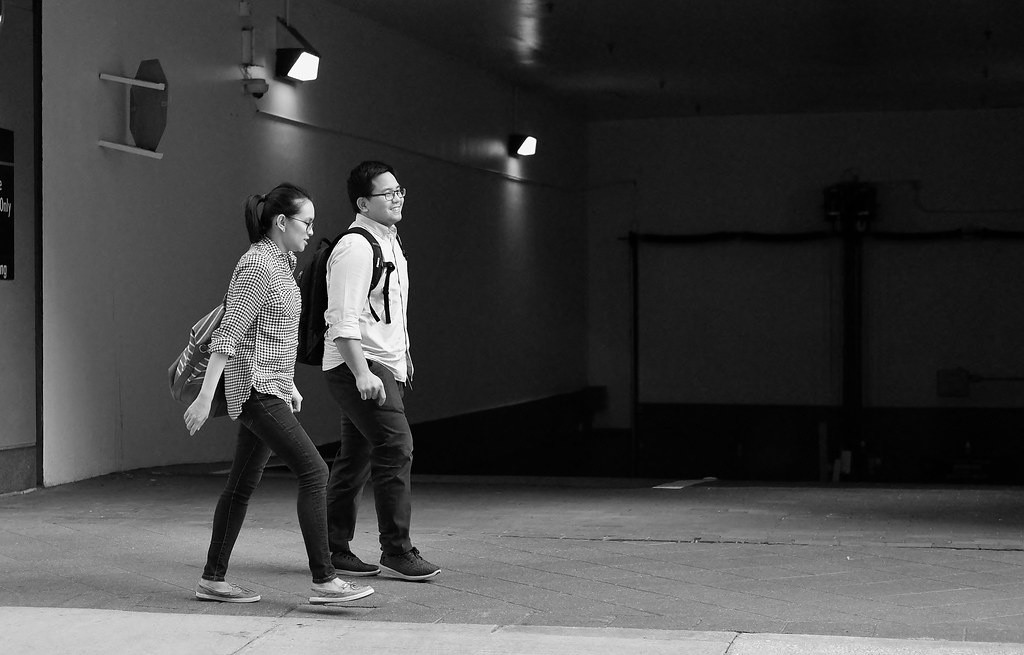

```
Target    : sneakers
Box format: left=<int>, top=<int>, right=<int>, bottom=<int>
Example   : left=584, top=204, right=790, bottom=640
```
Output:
left=379, top=547, right=441, bottom=581
left=331, top=549, right=381, bottom=577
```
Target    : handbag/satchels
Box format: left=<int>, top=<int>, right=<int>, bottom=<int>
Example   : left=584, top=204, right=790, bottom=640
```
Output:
left=168, top=293, right=229, bottom=418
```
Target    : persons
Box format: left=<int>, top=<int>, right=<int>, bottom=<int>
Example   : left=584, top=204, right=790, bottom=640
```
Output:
left=320, top=160, right=415, bottom=582
left=183, top=181, right=376, bottom=604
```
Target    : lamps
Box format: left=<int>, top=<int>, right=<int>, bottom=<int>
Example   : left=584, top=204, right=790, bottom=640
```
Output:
left=507, top=132, right=538, bottom=158
left=275, top=16, right=322, bottom=84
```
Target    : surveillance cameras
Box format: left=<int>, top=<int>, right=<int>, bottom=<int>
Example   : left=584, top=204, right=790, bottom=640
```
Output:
left=248, top=79, right=268, bottom=98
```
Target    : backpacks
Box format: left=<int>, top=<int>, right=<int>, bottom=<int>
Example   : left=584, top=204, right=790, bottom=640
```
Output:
left=297, top=228, right=395, bottom=366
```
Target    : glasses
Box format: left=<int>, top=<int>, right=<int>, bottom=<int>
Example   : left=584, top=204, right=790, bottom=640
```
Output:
left=287, top=216, right=315, bottom=232
left=363, top=187, right=407, bottom=200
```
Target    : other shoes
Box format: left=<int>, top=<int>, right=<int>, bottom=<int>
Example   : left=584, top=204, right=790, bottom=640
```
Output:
left=196, top=582, right=262, bottom=603
left=309, top=581, right=375, bottom=603
